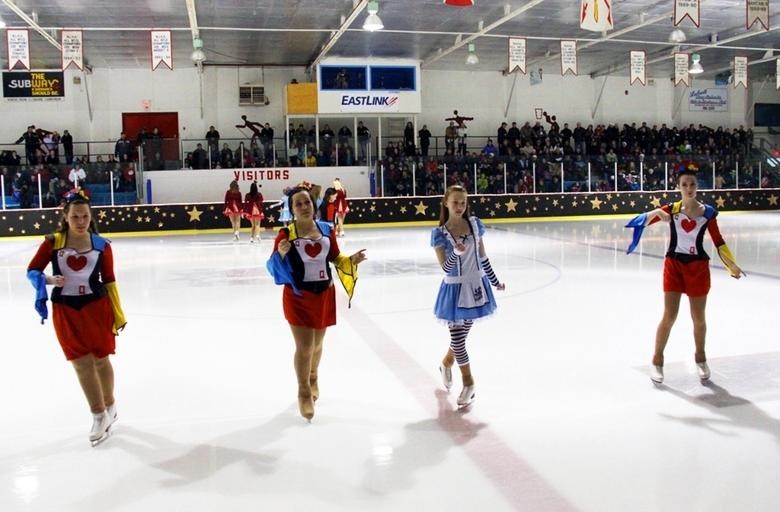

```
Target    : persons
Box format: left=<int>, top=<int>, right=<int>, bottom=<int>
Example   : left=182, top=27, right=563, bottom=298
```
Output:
left=297, top=124, right=308, bottom=163
left=331, top=181, right=351, bottom=235
left=733, top=128, right=740, bottom=155
left=562, top=141, right=575, bottom=155
left=419, top=125, right=432, bottom=157
left=514, top=139, right=521, bottom=150
left=308, top=126, right=321, bottom=159
left=586, top=125, right=594, bottom=155
left=652, top=124, right=658, bottom=155
left=321, top=124, right=335, bottom=161
left=69, top=163, right=87, bottom=192
left=235, top=141, right=249, bottom=168
left=60, top=130, right=73, bottom=163
left=506, top=122, right=520, bottom=147
left=536, top=140, right=546, bottom=155
left=27, top=194, right=127, bottom=441
left=631, top=122, right=638, bottom=155
left=671, top=127, right=680, bottom=155
left=243, top=183, right=265, bottom=242
left=520, top=142, right=534, bottom=156
left=97, top=156, right=105, bottom=163
left=697, top=124, right=708, bottom=154
left=14, top=127, right=40, bottom=166
left=560, top=123, right=573, bottom=155
left=253, top=143, right=265, bottom=168
left=497, top=122, right=508, bottom=155
left=152, top=128, right=164, bottom=159
left=206, top=126, right=220, bottom=169
left=520, top=142, right=536, bottom=158
left=244, top=150, right=256, bottom=167
left=547, top=125, right=565, bottom=155
left=623, top=123, right=630, bottom=156
left=663, top=137, right=673, bottom=155
left=501, top=138, right=519, bottom=164
left=266, top=186, right=293, bottom=228
left=540, top=138, right=555, bottom=155
left=520, top=121, right=537, bottom=142
left=606, top=149, right=618, bottom=163
left=382, top=156, right=780, bottom=197
left=338, top=126, right=354, bottom=161
left=680, top=127, right=689, bottom=146
left=193, top=143, right=207, bottom=169
left=223, top=180, right=243, bottom=241
left=594, top=125, right=602, bottom=155
left=638, top=122, right=656, bottom=155
left=724, top=128, right=732, bottom=155
left=318, top=188, right=339, bottom=233
left=688, top=124, right=697, bottom=151
left=658, top=124, right=672, bottom=156
left=405, top=122, right=415, bottom=157
left=746, top=128, right=754, bottom=156
left=482, top=139, right=497, bottom=155
left=115, top=132, right=134, bottom=162
left=107, top=155, right=118, bottom=162
left=0, top=150, right=73, bottom=208
left=445, top=121, right=456, bottom=157
left=185, top=152, right=193, bottom=169
left=284, top=123, right=297, bottom=162
left=81, top=155, right=90, bottom=163
left=614, top=123, right=620, bottom=156
left=609, top=122, right=616, bottom=156
left=32, top=125, right=53, bottom=164
left=52, top=132, right=60, bottom=163
left=396, top=141, right=405, bottom=156
left=599, top=124, right=607, bottom=156
left=532, top=122, right=547, bottom=155
left=261, top=122, right=274, bottom=167
left=572, top=122, right=586, bottom=155
left=456, top=123, right=469, bottom=157
left=266, top=187, right=368, bottom=419
left=386, top=142, right=395, bottom=159
left=739, top=125, right=747, bottom=155
left=151, top=153, right=166, bottom=170
left=135, top=127, right=151, bottom=156
left=220, top=143, right=236, bottom=167
left=430, top=186, right=506, bottom=405
left=713, top=127, right=723, bottom=154
left=43, top=133, right=56, bottom=163
left=300, top=182, right=322, bottom=221
left=624, top=171, right=747, bottom=383
left=357, top=121, right=371, bottom=162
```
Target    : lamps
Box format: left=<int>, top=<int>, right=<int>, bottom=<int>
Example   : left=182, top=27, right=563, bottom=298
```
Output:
left=670, top=23, right=686, bottom=44
left=192, top=36, right=206, bottom=61
left=688, top=52, right=704, bottom=73
left=466, top=39, right=479, bottom=65
left=362, top=1, right=383, bottom=31
left=0, top=15, right=6, bottom=30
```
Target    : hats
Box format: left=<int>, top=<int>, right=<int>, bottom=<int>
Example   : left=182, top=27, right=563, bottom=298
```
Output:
left=73, top=161, right=82, bottom=169
left=64, top=130, right=68, bottom=134
left=488, top=152, right=495, bottom=158
left=222, top=142, right=229, bottom=147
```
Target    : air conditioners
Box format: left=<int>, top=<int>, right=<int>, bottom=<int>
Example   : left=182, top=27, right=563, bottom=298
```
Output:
left=238, top=84, right=267, bottom=106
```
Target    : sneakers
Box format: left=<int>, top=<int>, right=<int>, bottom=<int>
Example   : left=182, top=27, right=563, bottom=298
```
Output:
left=233, top=236, right=236, bottom=241
left=309, top=376, right=321, bottom=402
left=234, top=231, right=240, bottom=240
left=340, top=231, right=345, bottom=238
left=107, top=403, right=120, bottom=431
left=694, top=356, right=711, bottom=381
left=249, top=238, right=255, bottom=244
left=335, top=230, right=339, bottom=236
left=87, top=408, right=115, bottom=442
left=456, top=382, right=478, bottom=406
left=255, top=234, right=262, bottom=242
left=647, top=360, right=663, bottom=384
left=440, top=362, right=454, bottom=391
left=297, top=392, right=315, bottom=420
